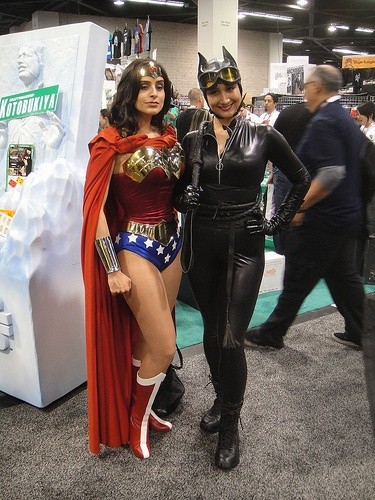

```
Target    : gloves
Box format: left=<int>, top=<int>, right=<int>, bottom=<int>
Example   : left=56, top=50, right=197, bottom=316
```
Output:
left=181, top=184, right=204, bottom=208
left=246, top=209, right=288, bottom=237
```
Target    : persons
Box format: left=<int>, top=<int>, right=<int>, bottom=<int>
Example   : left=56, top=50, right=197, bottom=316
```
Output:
left=1, top=39, right=66, bottom=177
left=163, top=97, right=179, bottom=128
left=81, top=58, right=186, bottom=461
left=356, top=100, right=375, bottom=147
left=98, top=107, right=113, bottom=133
left=239, top=101, right=259, bottom=124
left=166, top=45, right=311, bottom=470
left=258, top=91, right=280, bottom=128
left=176, top=87, right=212, bottom=142
left=242, top=65, right=365, bottom=350
left=272, top=99, right=315, bottom=255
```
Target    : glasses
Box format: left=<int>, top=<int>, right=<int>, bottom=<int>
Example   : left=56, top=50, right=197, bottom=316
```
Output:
left=299, top=81, right=318, bottom=91
left=199, top=68, right=242, bottom=89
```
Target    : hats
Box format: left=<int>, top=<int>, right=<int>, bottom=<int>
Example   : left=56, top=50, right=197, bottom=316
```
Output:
left=197, top=46, right=243, bottom=97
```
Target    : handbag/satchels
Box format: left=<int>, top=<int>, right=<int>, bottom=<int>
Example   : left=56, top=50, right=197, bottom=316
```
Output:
left=153, top=345, right=186, bottom=416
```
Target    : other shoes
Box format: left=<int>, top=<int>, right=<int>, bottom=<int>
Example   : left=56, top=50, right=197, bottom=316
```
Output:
left=243, top=327, right=282, bottom=349
left=332, top=331, right=361, bottom=348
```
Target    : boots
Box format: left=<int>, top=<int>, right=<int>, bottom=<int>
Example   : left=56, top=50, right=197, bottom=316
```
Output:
left=215, top=400, right=244, bottom=468
left=129, top=354, right=172, bottom=459
left=200, top=374, right=223, bottom=433
